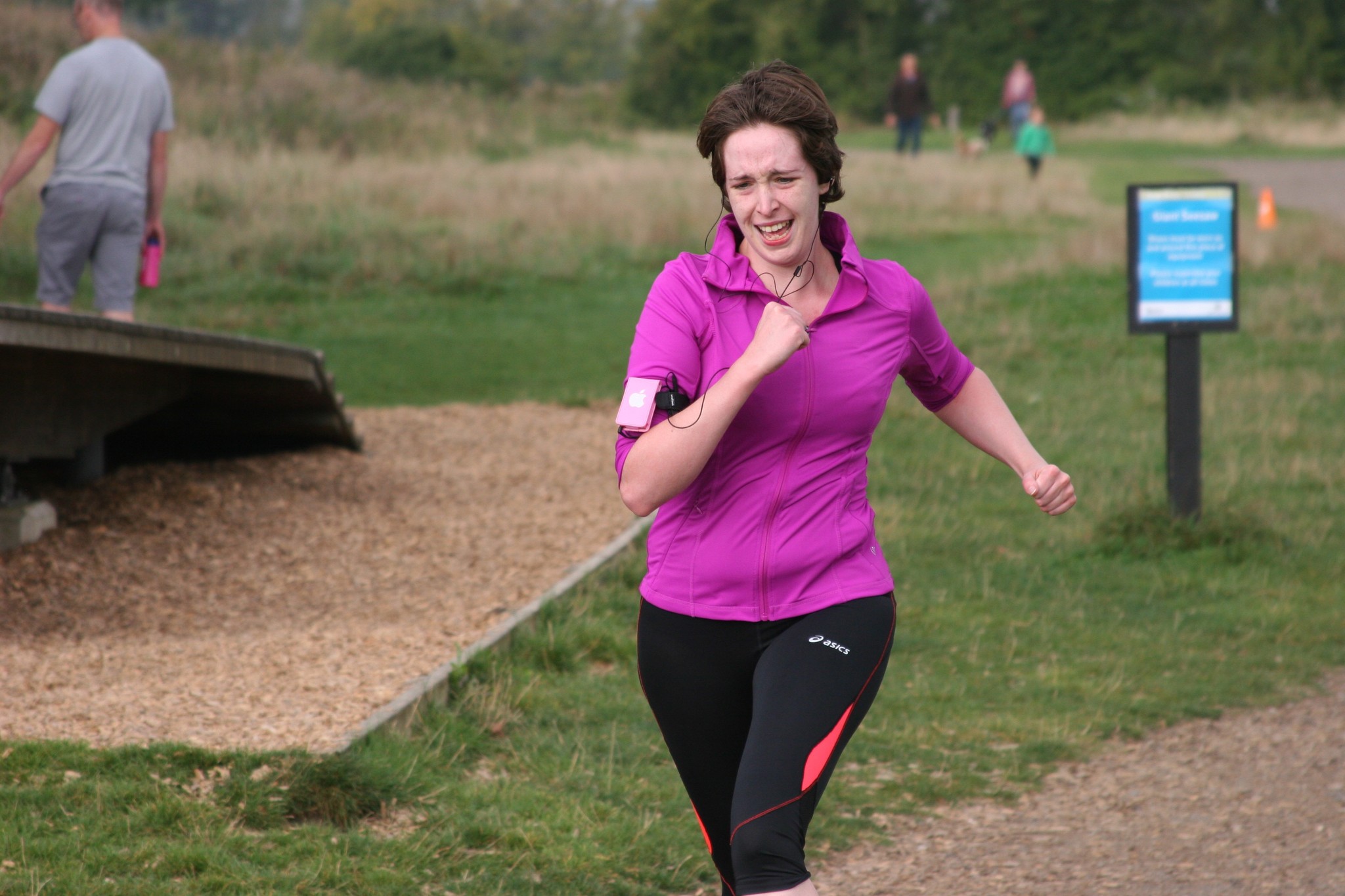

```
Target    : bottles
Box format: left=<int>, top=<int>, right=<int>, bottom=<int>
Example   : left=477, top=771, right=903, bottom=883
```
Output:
left=140, top=235, right=160, bottom=288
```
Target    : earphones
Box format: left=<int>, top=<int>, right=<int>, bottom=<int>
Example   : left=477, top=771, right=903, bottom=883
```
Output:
left=829, top=175, right=834, bottom=189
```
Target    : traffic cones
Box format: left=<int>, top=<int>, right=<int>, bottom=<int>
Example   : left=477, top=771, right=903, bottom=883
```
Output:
left=1255, top=185, right=1277, bottom=230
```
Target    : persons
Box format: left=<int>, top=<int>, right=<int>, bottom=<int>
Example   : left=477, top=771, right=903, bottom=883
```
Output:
left=1002, top=57, right=1055, bottom=179
left=886, top=54, right=939, bottom=155
left=0, top=1, right=174, bottom=325
left=612, top=59, right=1077, bottom=896
